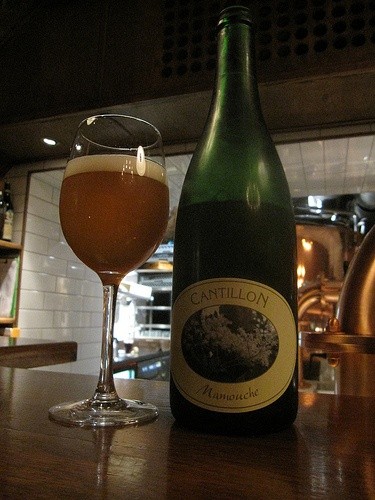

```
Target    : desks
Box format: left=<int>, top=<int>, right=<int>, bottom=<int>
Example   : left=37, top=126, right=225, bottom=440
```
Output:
left=0, top=337, right=375, bottom=500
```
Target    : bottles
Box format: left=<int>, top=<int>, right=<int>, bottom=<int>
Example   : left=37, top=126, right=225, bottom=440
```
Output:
left=170, top=5, right=299, bottom=439
left=0, top=182, right=15, bottom=243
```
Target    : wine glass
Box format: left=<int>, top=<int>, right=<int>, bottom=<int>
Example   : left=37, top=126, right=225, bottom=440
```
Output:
left=47, top=113, right=169, bottom=427
